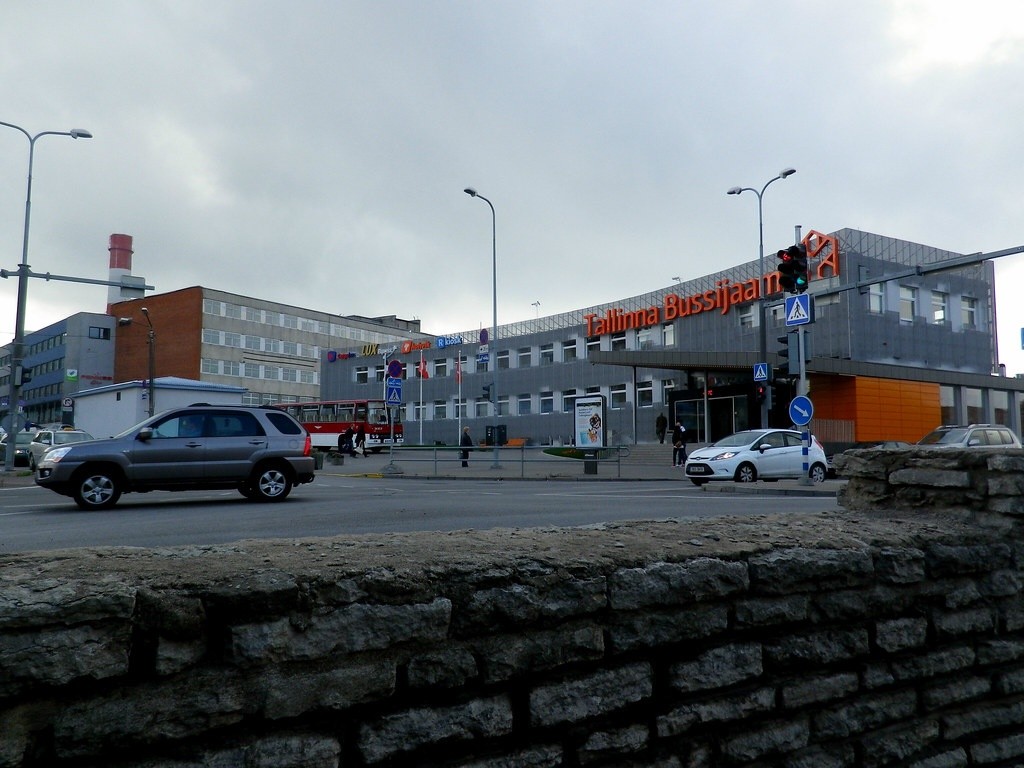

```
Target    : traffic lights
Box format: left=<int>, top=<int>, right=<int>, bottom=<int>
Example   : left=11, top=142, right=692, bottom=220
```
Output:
left=777, top=331, right=801, bottom=377
left=482, top=385, right=495, bottom=403
left=756, top=386, right=766, bottom=402
left=776, top=244, right=808, bottom=292
left=13, top=364, right=32, bottom=387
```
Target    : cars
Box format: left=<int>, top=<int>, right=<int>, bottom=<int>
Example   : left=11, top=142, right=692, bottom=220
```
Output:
left=0, top=423, right=70, bottom=466
left=685, top=430, right=828, bottom=486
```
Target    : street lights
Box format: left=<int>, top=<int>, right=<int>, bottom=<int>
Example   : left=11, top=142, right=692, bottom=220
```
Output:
left=1, top=120, right=92, bottom=469
left=464, top=188, right=500, bottom=469
left=726, top=165, right=798, bottom=430
left=120, top=308, right=155, bottom=416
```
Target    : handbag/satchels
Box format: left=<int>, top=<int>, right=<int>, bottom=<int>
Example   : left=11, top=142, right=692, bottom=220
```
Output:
left=675, top=441, right=682, bottom=448
left=354, top=441, right=364, bottom=455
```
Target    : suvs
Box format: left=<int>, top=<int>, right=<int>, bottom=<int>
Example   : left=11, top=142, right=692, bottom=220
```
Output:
left=35, top=402, right=317, bottom=510
left=29, top=426, right=95, bottom=468
left=915, top=423, right=1023, bottom=449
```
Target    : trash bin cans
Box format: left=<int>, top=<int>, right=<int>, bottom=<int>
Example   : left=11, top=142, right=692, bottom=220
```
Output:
left=485, top=426, right=496, bottom=446
left=497, top=424, right=508, bottom=445
left=584, top=453, right=597, bottom=475
left=313, top=453, right=324, bottom=470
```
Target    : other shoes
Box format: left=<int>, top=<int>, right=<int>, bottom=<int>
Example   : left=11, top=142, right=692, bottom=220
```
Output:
left=365, top=456, right=369, bottom=458
left=676, top=464, right=682, bottom=466
left=350, top=454, right=358, bottom=458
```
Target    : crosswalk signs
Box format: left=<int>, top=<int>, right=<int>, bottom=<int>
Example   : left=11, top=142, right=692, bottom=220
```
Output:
left=753, top=364, right=767, bottom=381
left=783, top=294, right=810, bottom=326
left=387, top=388, right=402, bottom=406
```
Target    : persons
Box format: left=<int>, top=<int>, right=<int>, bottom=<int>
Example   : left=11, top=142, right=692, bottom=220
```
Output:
left=345, top=425, right=369, bottom=458
left=656, top=413, right=667, bottom=444
left=672, top=422, right=687, bottom=467
left=460, top=426, right=473, bottom=467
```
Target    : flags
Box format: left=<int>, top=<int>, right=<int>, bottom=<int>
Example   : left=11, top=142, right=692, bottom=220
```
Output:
left=418, top=355, right=429, bottom=379
left=455, top=360, right=463, bottom=384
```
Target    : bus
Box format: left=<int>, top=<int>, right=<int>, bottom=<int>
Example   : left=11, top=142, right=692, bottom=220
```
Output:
left=273, top=400, right=405, bottom=453
